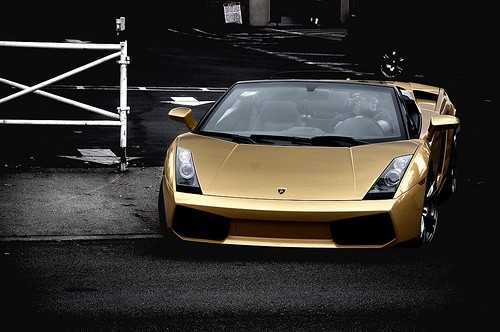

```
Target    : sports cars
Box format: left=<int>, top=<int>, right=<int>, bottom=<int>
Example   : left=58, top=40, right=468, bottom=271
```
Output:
left=158, top=78, right=462, bottom=265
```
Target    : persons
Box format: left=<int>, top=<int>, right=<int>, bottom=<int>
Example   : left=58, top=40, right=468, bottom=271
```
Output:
left=325, top=80, right=384, bottom=138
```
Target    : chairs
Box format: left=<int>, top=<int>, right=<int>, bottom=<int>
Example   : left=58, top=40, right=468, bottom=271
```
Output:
left=251, top=90, right=397, bottom=136
